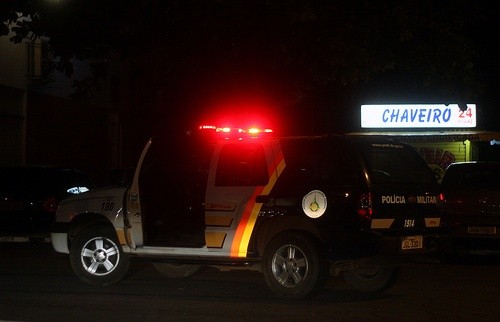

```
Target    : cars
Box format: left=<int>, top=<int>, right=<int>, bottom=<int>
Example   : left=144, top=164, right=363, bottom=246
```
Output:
left=440, top=159, right=500, bottom=266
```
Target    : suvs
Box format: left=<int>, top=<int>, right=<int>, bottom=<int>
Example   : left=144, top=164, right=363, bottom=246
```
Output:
left=49, top=124, right=445, bottom=302
